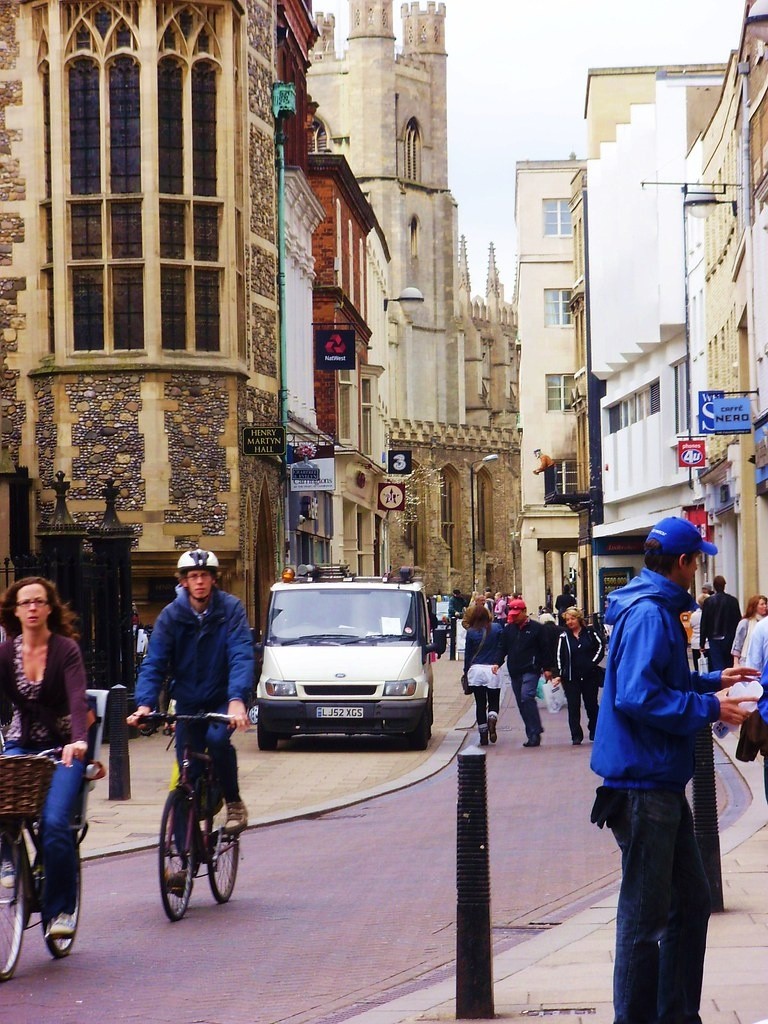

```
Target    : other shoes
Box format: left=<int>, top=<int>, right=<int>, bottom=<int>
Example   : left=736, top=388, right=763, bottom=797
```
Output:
left=573, top=739, right=581, bottom=744
left=168, top=870, right=193, bottom=898
left=488, top=715, right=497, bottom=743
left=480, top=732, right=488, bottom=745
left=49, top=913, right=76, bottom=937
left=225, top=801, right=248, bottom=834
left=1, top=860, right=16, bottom=888
left=590, top=732, right=594, bottom=740
left=523, top=734, right=540, bottom=747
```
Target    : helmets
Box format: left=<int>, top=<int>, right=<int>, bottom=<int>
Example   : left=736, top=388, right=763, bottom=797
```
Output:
left=177, top=549, right=219, bottom=568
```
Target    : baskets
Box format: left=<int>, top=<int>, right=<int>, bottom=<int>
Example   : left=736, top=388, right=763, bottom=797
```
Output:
left=0, top=754, right=53, bottom=815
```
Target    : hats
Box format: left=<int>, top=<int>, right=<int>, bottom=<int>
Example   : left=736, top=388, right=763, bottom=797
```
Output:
left=702, top=583, right=711, bottom=590
left=508, top=600, right=525, bottom=615
left=644, top=516, right=717, bottom=555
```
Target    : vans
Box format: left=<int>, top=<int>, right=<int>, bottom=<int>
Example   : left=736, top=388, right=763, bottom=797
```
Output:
left=254, top=561, right=443, bottom=752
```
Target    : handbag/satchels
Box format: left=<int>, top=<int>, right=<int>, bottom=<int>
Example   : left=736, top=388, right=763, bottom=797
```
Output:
left=536, top=676, right=567, bottom=713
left=698, top=652, right=708, bottom=676
left=461, top=675, right=473, bottom=695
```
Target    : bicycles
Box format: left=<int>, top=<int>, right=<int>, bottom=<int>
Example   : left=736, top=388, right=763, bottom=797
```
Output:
left=134, top=713, right=243, bottom=923
left=0, top=727, right=90, bottom=981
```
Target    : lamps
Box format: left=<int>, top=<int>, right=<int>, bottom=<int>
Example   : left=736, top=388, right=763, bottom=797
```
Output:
left=383, top=286, right=425, bottom=319
left=683, top=186, right=738, bottom=219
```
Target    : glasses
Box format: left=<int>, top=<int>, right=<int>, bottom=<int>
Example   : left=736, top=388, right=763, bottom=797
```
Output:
left=16, top=600, right=50, bottom=606
left=508, top=606, right=526, bottom=610
left=186, top=573, right=209, bottom=578
left=566, top=607, right=577, bottom=611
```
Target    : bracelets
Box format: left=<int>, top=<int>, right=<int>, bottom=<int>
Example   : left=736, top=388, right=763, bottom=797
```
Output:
left=76, top=741, right=87, bottom=743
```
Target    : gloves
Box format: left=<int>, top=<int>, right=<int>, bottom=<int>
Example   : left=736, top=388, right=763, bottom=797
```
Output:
left=590, top=787, right=628, bottom=831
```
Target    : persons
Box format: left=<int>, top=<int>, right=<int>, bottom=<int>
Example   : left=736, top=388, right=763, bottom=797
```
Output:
left=532, top=448, right=560, bottom=492
left=126, top=549, right=256, bottom=898
left=0, top=576, right=89, bottom=936
left=463, top=605, right=504, bottom=748
left=555, top=584, right=577, bottom=626
left=744, top=614, right=768, bottom=805
left=448, top=586, right=523, bottom=630
left=590, top=517, right=761, bottom=1024
left=491, top=599, right=605, bottom=747
left=690, top=575, right=768, bottom=673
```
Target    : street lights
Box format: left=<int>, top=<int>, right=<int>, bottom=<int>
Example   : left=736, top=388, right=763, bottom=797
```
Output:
left=470, top=452, right=499, bottom=592
